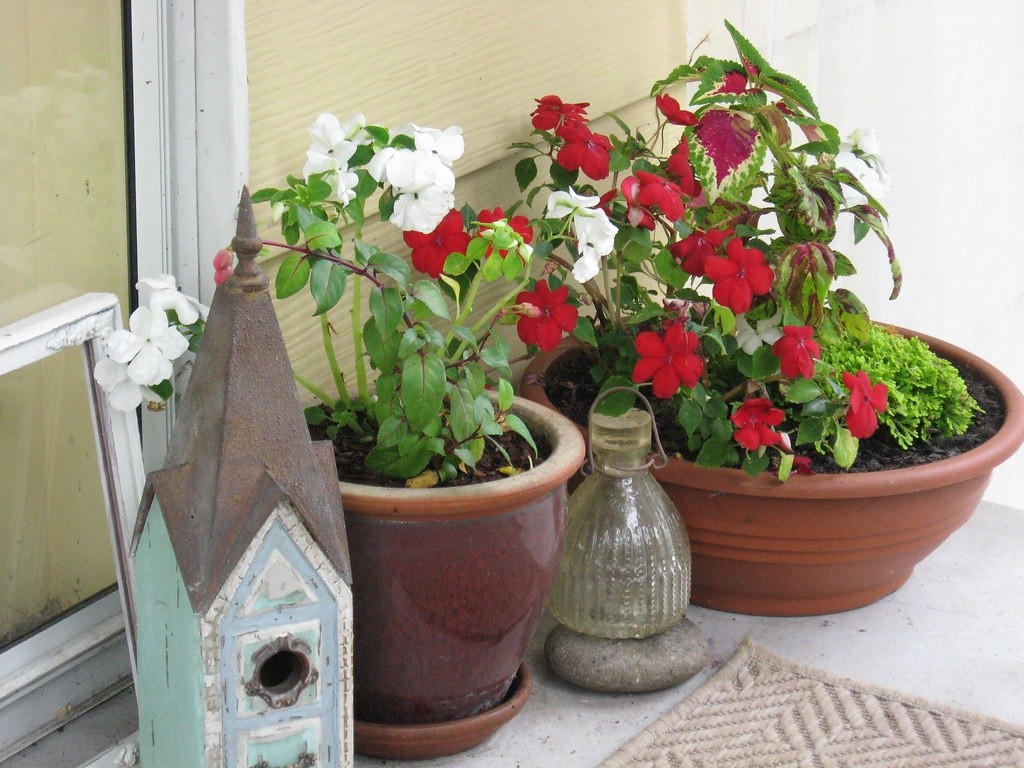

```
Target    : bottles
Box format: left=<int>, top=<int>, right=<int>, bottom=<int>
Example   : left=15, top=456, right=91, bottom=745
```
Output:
left=545, top=385, right=693, bottom=638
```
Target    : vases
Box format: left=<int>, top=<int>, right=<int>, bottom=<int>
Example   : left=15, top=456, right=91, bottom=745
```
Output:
left=285, top=390, right=587, bottom=759
left=522, top=314, right=1024, bottom=617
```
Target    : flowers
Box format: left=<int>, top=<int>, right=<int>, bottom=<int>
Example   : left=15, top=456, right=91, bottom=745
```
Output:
left=92, top=113, right=619, bottom=483
left=401, top=19, right=989, bottom=482
